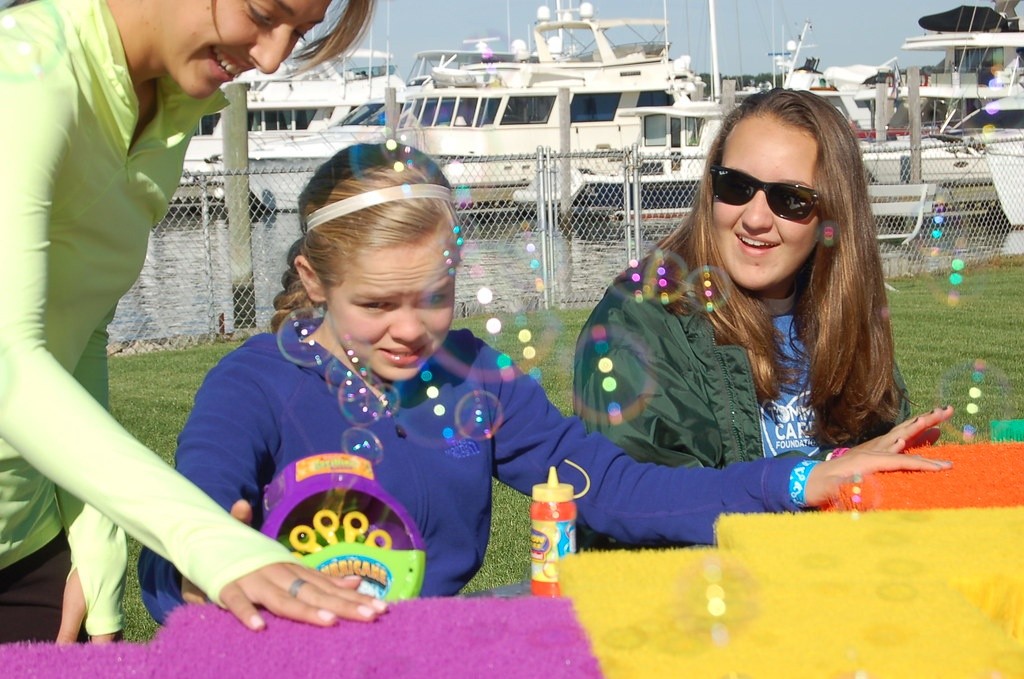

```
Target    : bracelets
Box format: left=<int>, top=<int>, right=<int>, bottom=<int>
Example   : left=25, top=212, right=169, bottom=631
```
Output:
left=789, top=460, right=821, bottom=509
left=830, top=448, right=850, bottom=460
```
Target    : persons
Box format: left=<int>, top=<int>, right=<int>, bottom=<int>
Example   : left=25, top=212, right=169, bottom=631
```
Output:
left=0, top=0, right=387, bottom=646
left=480, top=46, right=493, bottom=63
left=137, top=143, right=949, bottom=628
left=576, top=87, right=953, bottom=548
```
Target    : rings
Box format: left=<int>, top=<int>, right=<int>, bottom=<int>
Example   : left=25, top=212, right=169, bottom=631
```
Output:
left=287, top=578, right=305, bottom=597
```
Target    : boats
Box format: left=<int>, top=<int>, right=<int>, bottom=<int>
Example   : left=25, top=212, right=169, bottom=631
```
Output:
left=166, top=0, right=1024, bottom=235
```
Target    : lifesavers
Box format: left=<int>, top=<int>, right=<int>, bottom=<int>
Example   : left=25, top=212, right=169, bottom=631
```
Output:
left=884, top=73, right=894, bottom=87
left=920, top=71, right=928, bottom=86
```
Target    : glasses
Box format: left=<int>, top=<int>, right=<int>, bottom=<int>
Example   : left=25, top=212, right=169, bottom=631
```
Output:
left=710, top=165, right=818, bottom=219
